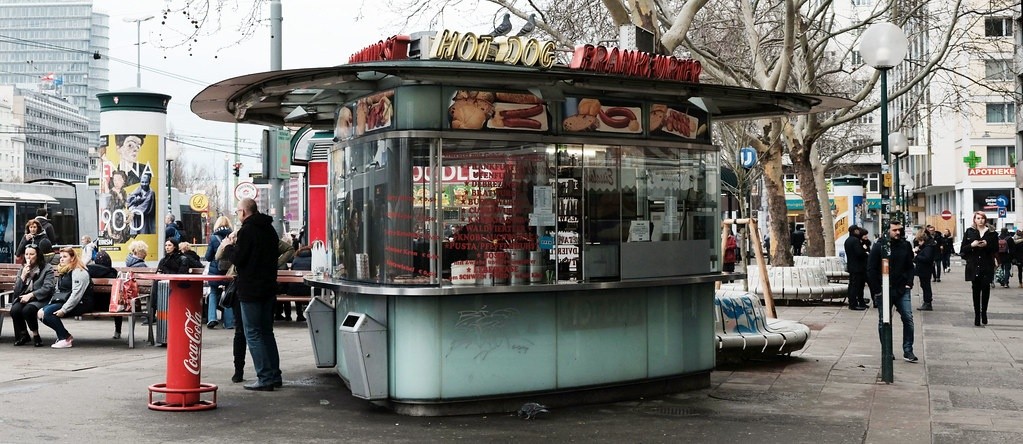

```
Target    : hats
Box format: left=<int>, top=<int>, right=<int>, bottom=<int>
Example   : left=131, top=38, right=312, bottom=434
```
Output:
left=1016, top=229, right=1021, bottom=236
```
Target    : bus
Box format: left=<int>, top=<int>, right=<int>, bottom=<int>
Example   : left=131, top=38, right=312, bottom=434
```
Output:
left=0, top=178, right=209, bottom=265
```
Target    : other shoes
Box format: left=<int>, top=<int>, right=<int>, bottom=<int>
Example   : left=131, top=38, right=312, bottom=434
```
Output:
left=975, top=280, right=1023, bottom=327
left=207, top=321, right=219, bottom=329
left=141, top=318, right=157, bottom=326
left=932, top=266, right=951, bottom=282
left=113, top=332, right=121, bottom=339
left=232, top=375, right=244, bottom=383
left=275, top=315, right=306, bottom=323
left=850, top=298, right=870, bottom=310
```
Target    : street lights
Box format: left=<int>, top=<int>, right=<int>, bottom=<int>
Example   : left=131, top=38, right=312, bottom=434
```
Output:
left=858, top=23, right=907, bottom=383
left=166, top=142, right=180, bottom=212
left=122, top=15, right=155, bottom=90
left=888, top=132, right=914, bottom=239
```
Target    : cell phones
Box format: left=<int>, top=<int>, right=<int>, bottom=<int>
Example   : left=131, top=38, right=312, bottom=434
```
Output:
left=979, top=242, right=984, bottom=244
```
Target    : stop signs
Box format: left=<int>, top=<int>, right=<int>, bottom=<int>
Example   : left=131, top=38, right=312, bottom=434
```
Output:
left=942, top=210, right=951, bottom=220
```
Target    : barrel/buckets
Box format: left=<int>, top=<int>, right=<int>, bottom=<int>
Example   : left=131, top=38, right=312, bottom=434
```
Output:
left=485, top=252, right=506, bottom=283
left=529, top=251, right=543, bottom=282
left=503, top=248, right=530, bottom=285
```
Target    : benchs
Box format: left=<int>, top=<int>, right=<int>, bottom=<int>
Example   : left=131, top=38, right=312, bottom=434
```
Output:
left=792, top=255, right=850, bottom=282
left=0, top=261, right=334, bottom=349
left=721, top=264, right=848, bottom=306
left=714, top=290, right=811, bottom=365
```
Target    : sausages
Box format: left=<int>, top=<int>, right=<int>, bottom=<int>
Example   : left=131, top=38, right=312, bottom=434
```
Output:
left=499, top=105, right=544, bottom=129
left=597, top=107, right=637, bottom=128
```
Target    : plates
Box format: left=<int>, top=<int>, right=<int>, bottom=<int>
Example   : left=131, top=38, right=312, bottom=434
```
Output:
left=365, top=102, right=391, bottom=131
left=487, top=102, right=547, bottom=130
left=662, top=109, right=699, bottom=139
left=596, top=105, right=643, bottom=133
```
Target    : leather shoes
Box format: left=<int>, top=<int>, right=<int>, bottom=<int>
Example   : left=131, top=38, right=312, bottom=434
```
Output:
left=274, top=380, right=282, bottom=390
left=243, top=380, right=274, bottom=391
left=14, top=334, right=43, bottom=347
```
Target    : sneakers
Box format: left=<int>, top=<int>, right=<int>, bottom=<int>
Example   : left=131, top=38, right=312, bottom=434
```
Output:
left=893, top=351, right=918, bottom=362
left=51, top=335, right=74, bottom=348
left=916, top=302, right=933, bottom=311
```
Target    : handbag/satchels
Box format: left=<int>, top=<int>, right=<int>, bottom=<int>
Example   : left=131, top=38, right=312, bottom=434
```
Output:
left=51, top=290, right=72, bottom=303
left=311, top=240, right=329, bottom=276
left=109, top=271, right=142, bottom=313
left=965, top=262, right=973, bottom=281
left=169, top=226, right=181, bottom=243
left=219, top=277, right=241, bottom=309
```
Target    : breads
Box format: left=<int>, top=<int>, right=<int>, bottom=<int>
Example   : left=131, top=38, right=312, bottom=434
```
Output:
left=336, top=105, right=366, bottom=138
left=562, top=99, right=600, bottom=132
left=649, top=105, right=669, bottom=132
left=448, top=90, right=495, bottom=129
left=496, top=93, right=543, bottom=104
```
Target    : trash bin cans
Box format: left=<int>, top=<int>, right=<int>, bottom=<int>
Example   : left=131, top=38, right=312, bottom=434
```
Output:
left=339, top=312, right=388, bottom=400
left=304, top=296, right=337, bottom=368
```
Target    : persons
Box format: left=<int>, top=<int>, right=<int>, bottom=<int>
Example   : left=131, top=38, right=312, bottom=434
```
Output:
left=277, top=234, right=311, bottom=321
left=844, top=225, right=871, bottom=310
left=448, top=199, right=506, bottom=261
left=874, top=233, right=879, bottom=243
left=722, top=226, right=745, bottom=283
left=960, top=211, right=998, bottom=325
left=913, top=225, right=954, bottom=310
left=9, top=208, right=148, bottom=349
left=140, top=215, right=236, bottom=328
left=222, top=197, right=283, bottom=390
left=867, top=220, right=918, bottom=363
left=791, top=224, right=805, bottom=255
left=985, top=223, right=1023, bottom=287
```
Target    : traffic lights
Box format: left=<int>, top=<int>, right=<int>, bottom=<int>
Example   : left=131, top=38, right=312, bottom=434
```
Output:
left=233, top=163, right=239, bottom=176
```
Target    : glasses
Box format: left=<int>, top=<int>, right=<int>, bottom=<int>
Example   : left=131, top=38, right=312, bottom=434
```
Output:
left=234, top=209, right=242, bottom=215
left=25, top=244, right=38, bottom=248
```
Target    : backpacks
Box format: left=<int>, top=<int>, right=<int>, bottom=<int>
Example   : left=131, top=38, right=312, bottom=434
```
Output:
left=213, top=234, right=234, bottom=271
left=186, top=254, right=205, bottom=274
left=998, top=235, right=1011, bottom=252
